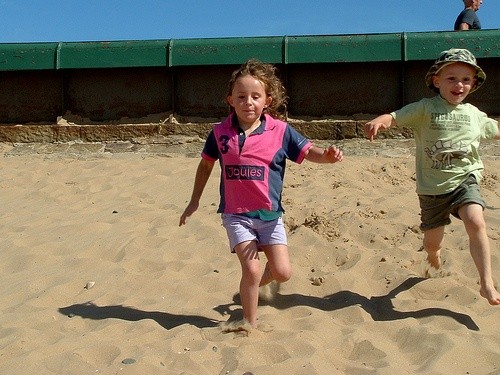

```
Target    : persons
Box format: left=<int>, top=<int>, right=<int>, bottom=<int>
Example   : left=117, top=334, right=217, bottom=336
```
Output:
left=363, top=49, right=500, bottom=306
left=179, top=59, right=344, bottom=328
left=454, top=0, right=483, bottom=31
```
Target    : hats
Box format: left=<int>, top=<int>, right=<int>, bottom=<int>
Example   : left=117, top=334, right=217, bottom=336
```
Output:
left=423, top=48, right=487, bottom=95
